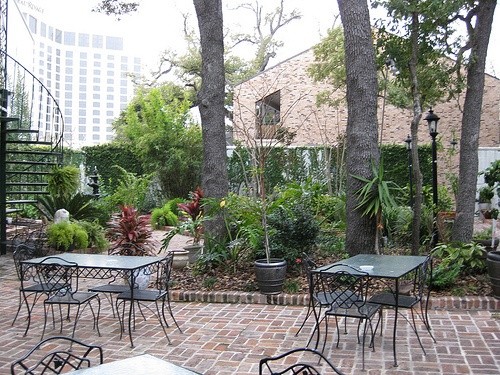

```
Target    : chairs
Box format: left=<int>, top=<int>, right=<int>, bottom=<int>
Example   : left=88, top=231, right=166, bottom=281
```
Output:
left=10, top=244, right=183, bottom=375
left=259, top=252, right=438, bottom=375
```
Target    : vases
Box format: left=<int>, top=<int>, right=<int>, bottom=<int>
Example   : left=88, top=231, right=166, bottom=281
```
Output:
left=254, top=257, right=286, bottom=295
left=167, top=250, right=189, bottom=270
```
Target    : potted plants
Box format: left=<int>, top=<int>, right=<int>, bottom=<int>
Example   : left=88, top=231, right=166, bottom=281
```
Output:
left=177, top=186, right=205, bottom=263
left=479, top=159, right=500, bottom=299
left=105, top=203, right=161, bottom=289
left=435, top=186, right=456, bottom=243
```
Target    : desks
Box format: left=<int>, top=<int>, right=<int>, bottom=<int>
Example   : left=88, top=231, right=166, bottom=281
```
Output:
left=55, top=353, right=205, bottom=375
left=20, top=253, right=164, bottom=349
left=310, top=254, right=431, bottom=367
